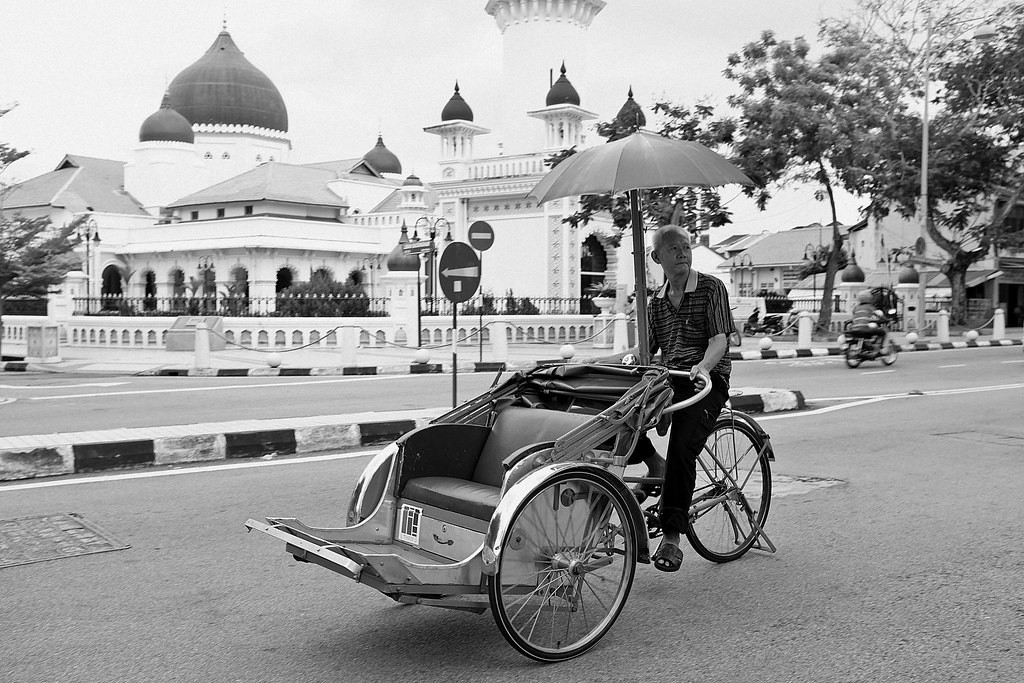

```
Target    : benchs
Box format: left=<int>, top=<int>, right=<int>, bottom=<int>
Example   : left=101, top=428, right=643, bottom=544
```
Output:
left=399, top=405, right=599, bottom=563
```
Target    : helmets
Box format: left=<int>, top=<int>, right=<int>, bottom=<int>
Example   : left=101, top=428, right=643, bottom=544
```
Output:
left=859, top=291, right=874, bottom=304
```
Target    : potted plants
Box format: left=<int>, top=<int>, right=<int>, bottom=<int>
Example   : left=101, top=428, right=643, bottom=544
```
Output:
left=586, top=281, right=616, bottom=307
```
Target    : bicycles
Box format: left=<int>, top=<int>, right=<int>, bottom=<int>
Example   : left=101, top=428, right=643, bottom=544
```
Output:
left=728, top=306, right=741, bottom=347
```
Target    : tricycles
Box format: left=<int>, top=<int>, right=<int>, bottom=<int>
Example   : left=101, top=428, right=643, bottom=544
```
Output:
left=244, top=352, right=778, bottom=663
left=837, top=286, right=905, bottom=369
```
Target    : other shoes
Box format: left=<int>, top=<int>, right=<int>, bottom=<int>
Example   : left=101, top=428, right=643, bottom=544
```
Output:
left=880, top=348, right=890, bottom=355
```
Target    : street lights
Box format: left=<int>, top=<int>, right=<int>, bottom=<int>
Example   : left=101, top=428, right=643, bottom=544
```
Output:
left=409, top=217, right=455, bottom=316
left=731, top=253, right=754, bottom=297
left=802, top=242, right=828, bottom=314
left=71, top=220, right=102, bottom=316
left=913, top=24, right=996, bottom=339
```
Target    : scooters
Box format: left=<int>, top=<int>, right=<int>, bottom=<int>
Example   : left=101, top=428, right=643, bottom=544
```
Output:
left=743, top=306, right=784, bottom=338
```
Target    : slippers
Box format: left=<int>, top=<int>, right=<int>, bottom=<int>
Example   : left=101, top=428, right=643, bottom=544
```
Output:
left=630, top=476, right=653, bottom=504
left=655, top=543, right=683, bottom=571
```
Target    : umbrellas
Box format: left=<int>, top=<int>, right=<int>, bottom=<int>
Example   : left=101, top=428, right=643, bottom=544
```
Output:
left=526, top=112, right=757, bottom=211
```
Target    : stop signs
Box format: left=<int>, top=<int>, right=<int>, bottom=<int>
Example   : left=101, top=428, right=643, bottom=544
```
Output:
left=468, top=221, right=496, bottom=251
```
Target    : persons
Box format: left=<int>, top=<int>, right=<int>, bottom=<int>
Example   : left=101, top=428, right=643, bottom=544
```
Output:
left=608, top=224, right=736, bottom=573
left=852, top=292, right=888, bottom=354
